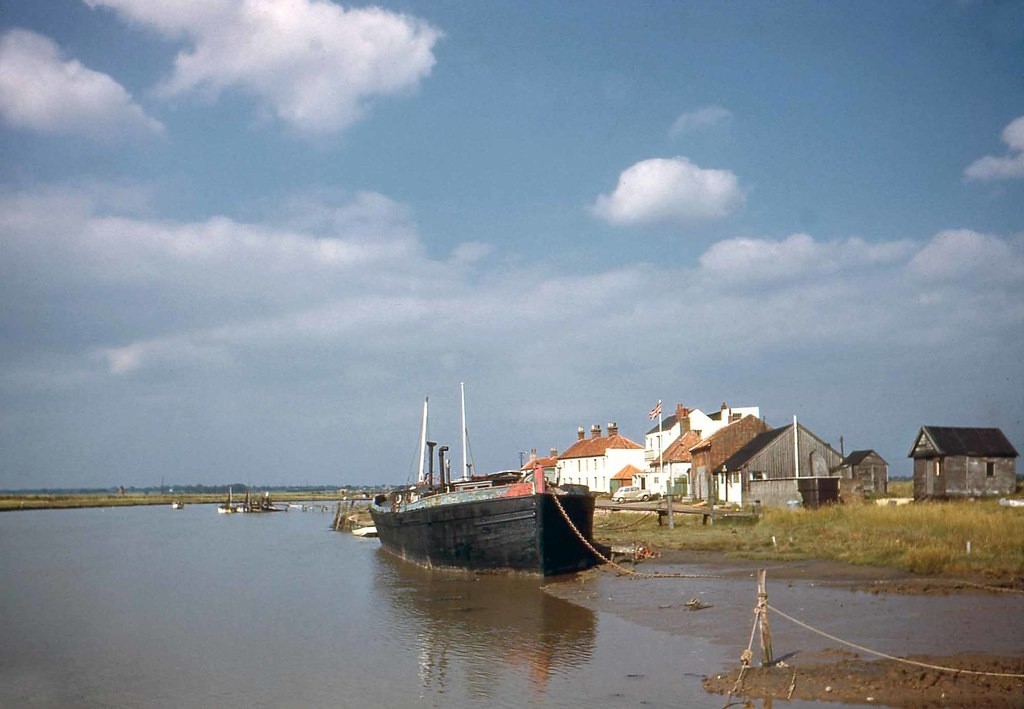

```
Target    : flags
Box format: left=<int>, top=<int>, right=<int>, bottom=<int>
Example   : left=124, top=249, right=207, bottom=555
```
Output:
left=649, top=403, right=662, bottom=421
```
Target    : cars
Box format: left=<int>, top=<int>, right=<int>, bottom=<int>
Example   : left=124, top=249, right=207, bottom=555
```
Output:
left=611, top=486, right=652, bottom=504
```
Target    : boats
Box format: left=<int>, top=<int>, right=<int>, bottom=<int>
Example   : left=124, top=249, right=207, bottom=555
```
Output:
left=370, top=381, right=598, bottom=580
